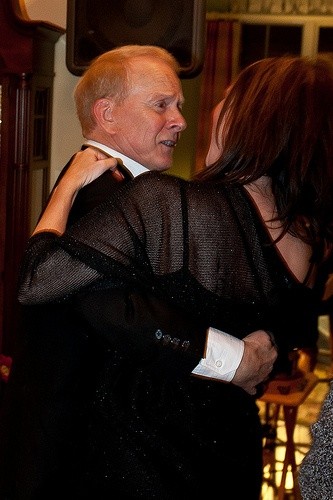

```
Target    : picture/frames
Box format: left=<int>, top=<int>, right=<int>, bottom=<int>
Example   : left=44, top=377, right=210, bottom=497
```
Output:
left=64, top=0, right=203, bottom=78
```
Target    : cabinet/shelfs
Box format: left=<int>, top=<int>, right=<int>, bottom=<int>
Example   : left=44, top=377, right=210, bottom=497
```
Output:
left=0, top=0, right=67, bottom=356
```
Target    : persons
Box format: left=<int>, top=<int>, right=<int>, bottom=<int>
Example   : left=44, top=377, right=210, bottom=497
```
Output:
left=0, top=45, right=277, bottom=500
left=15, top=51, right=333, bottom=500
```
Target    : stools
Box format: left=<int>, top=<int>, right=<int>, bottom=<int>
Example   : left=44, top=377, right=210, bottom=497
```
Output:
left=258, top=371, right=320, bottom=500
